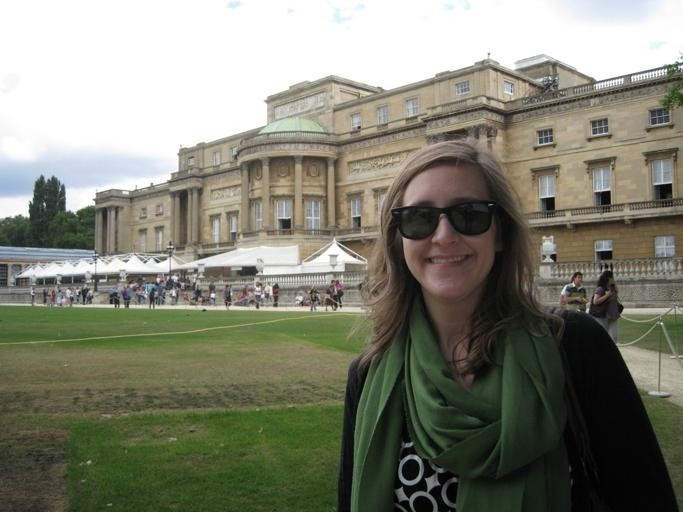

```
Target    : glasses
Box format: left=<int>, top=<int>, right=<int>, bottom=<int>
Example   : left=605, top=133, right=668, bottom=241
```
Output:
left=392, top=198, right=497, bottom=239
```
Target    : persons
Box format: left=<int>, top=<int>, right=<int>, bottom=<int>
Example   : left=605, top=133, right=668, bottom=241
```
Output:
left=31, top=284, right=94, bottom=309
left=223, top=281, right=281, bottom=309
left=591, top=271, right=624, bottom=346
left=340, top=140, right=677, bottom=510
left=109, top=280, right=216, bottom=309
left=557, top=273, right=576, bottom=306
left=563, top=272, right=590, bottom=312
left=295, top=280, right=344, bottom=310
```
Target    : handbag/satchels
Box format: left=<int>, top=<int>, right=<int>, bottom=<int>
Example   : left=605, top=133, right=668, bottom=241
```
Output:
left=589, top=295, right=607, bottom=318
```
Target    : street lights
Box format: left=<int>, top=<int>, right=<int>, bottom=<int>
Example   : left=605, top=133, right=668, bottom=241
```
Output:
left=163, top=240, right=175, bottom=305
left=92, top=247, right=100, bottom=292
